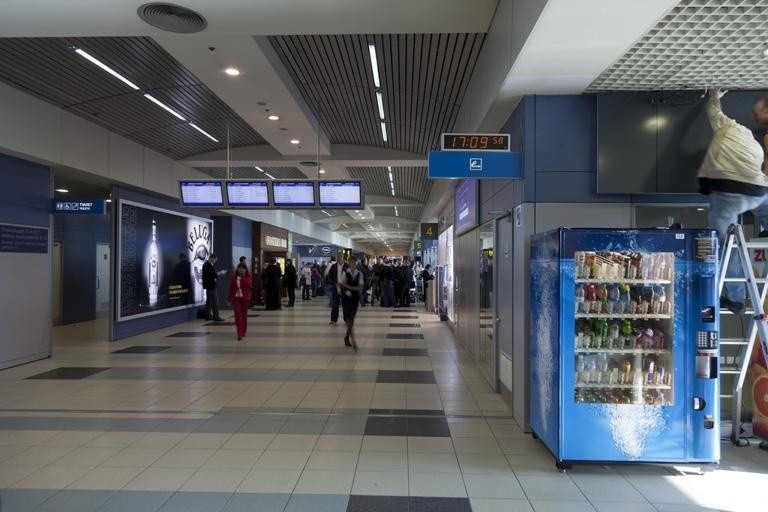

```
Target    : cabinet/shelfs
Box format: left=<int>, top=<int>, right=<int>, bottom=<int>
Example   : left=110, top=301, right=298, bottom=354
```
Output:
left=575, top=277, right=672, bottom=391
left=630, top=203, right=768, bottom=441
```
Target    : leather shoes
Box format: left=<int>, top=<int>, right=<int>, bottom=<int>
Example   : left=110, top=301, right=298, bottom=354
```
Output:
left=206, top=315, right=224, bottom=321
left=352, top=344, right=358, bottom=351
left=343, top=336, right=351, bottom=346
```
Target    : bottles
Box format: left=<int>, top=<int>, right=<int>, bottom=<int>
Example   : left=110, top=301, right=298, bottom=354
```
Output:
left=576, top=252, right=674, bottom=405
left=145, top=220, right=160, bottom=304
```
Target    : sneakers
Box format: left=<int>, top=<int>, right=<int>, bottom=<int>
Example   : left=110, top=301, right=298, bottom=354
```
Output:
left=329, top=321, right=335, bottom=325
left=302, top=296, right=312, bottom=300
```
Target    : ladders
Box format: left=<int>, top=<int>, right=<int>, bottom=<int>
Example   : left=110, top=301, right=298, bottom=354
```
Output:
left=718, top=224, right=768, bottom=450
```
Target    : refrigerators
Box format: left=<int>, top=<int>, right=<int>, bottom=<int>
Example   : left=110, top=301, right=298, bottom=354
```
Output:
left=528, top=225, right=722, bottom=468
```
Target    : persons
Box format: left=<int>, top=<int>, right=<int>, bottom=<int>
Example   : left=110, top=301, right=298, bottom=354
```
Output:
left=202, top=253, right=436, bottom=353
left=696, top=85, right=768, bottom=315
left=753, top=97, right=768, bottom=174
left=174, top=253, right=190, bottom=304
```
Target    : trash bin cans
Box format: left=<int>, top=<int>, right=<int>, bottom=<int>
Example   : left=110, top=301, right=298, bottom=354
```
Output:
left=426, top=280, right=437, bottom=312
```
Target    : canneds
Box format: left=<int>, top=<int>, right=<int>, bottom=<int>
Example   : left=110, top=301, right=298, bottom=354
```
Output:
left=632, top=326, right=665, bottom=349
left=657, top=365, right=666, bottom=385
left=583, top=326, right=595, bottom=347
left=574, top=252, right=597, bottom=278
left=598, top=252, right=632, bottom=278
left=621, top=360, right=631, bottom=383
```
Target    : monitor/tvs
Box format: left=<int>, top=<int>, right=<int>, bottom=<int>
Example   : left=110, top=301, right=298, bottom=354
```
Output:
left=176, top=178, right=364, bottom=209
left=596, top=91, right=768, bottom=195
